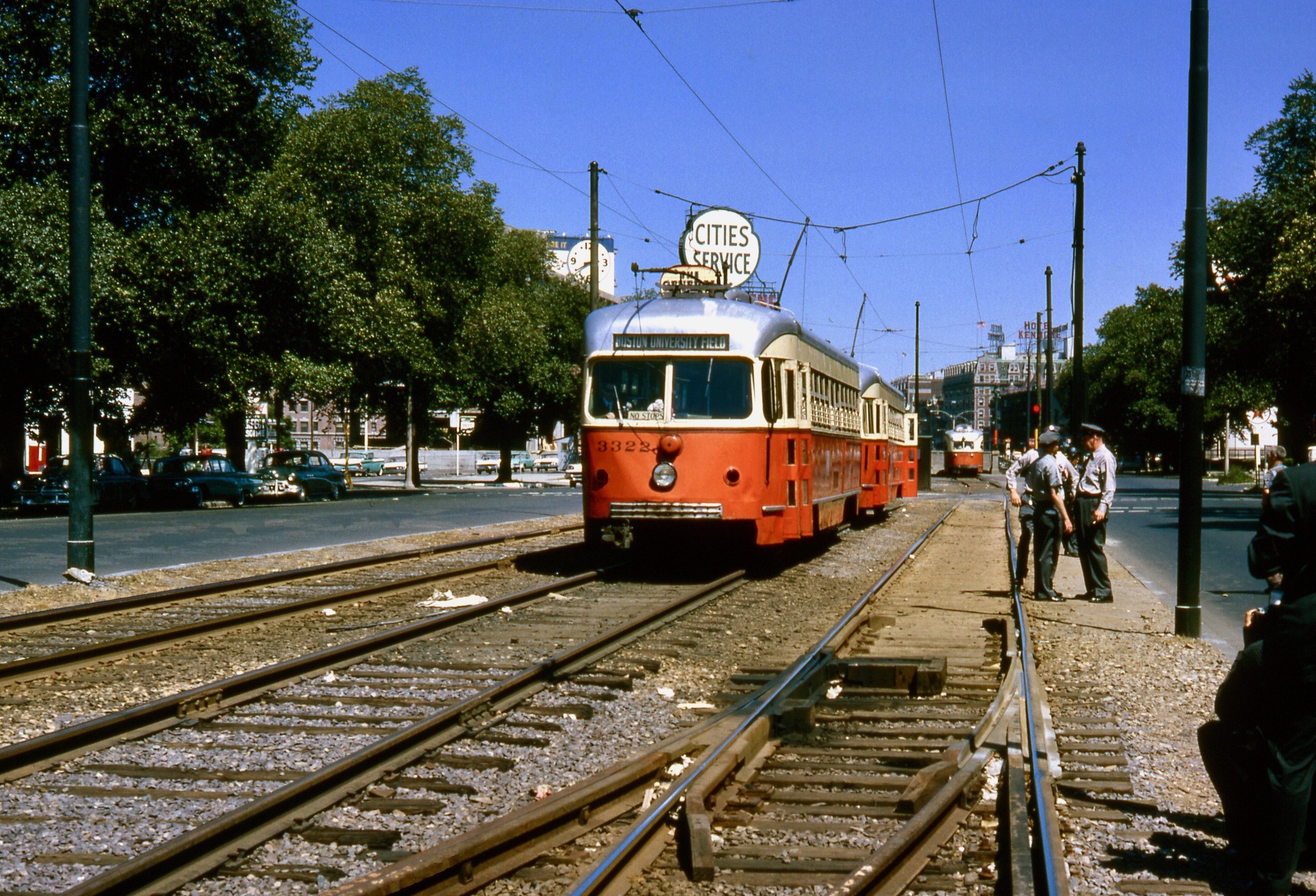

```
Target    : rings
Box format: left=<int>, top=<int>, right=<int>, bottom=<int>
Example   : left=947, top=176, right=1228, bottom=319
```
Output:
left=1068, top=528, right=1070, bottom=530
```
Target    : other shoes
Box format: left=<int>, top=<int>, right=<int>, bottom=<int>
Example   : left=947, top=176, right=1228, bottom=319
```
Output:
left=1064, top=551, right=1078, bottom=557
left=1034, top=592, right=1066, bottom=602
left=1014, top=577, right=1026, bottom=585
left=1077, top=592, right=1096, bottom=600
left=1090, top=594, right=1113, bottom=602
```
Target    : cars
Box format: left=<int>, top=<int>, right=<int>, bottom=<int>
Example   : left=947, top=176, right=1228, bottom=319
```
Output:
left=329, top=450, right=386, bottom=477
left=253, top=450, right=346, bottom=502
left=512, top=452, right=535, bottom=472
left=384, top=454, right=428, bottom=475
left=146, top=449, right=268, bottom=506
left=9, top=452, right=148, bottom=517
left=476, top=453, right=503, bottom=471
left=533, top=451, right=560, bottom=472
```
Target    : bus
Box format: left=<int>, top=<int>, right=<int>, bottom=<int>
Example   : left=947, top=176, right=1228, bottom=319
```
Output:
left=947, top=423, right=986, bottom=475
left=858, top=364, right=921, bottom=520
left=578, top=216, right=860, bottom=558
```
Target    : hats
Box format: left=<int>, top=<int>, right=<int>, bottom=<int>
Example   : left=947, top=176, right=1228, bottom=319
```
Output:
left=1043, top=425, right=1061, bottom=432
left=1081, top=422, right=1105, bottom=436
left=1039, top=432, right=1060, bottom=446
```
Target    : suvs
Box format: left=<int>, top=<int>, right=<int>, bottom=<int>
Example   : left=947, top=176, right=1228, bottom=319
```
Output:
left=564, top=453, right=583, bottom=488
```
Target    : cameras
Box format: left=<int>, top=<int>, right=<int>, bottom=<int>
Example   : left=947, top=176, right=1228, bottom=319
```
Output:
left=1252, top=592, right=1283, bottom=628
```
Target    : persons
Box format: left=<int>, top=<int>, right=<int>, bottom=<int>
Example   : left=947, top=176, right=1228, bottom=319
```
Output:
left=1027, top=431, right=1074, bottom=602
left=1193, top=460, right=1316, bottom=896
left=1063, top=447, right=1084, bottom=557
left=1261, top=447, right=1290, bottom=506
left=1075, top=422, right=1117, bottom=603
left=1240, top=570, right=1284, bottom=649
left=1005, top=425, right=1081, bottom=595
